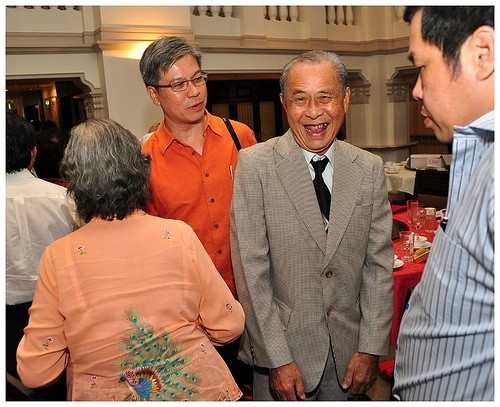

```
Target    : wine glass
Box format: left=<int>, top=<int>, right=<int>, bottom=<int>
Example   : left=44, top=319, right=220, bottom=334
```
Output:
left=412, top=207, right=424, bottom=242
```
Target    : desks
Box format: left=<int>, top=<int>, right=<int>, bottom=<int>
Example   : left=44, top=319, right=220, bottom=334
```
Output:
left=379, top=204, right=439, bottom=379
left=384, top=165, right=416, bottom=196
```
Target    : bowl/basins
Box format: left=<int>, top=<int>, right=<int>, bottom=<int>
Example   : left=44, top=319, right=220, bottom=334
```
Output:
left=414, top=236, right=428, bottom=246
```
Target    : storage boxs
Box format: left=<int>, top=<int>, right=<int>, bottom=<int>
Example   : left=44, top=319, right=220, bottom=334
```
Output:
left=411, top=154, right=452, bottom=170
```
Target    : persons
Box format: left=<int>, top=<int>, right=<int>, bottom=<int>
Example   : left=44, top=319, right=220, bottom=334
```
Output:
left=6, top=114, right=81, bottom=401
left=230, top=51, right=394, bottom=400
left=394, top=6, right=494, bottom=402
left=28, top=121, right=71, bottom=178
left=15, top=117, right=244, bottom=401
left=138, top=36, right=256, bottom=390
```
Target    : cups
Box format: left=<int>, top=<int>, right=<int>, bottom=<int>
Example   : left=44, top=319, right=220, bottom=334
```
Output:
left=406, top=200, right=418, bottom=223
left=422, top=209, right=436, bottom=233
left=385, top=160, right=409, bottom=172
left=399, top=231, right=415, bottom=263
left=413, top=248, right=431, bottom=263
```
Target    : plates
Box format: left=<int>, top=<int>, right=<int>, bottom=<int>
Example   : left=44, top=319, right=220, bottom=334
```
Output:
left=393, top=259, right=403, bottom=269
left=386, top=170, right=399, bottom=174
left=413, top=242, right=432, bottom=249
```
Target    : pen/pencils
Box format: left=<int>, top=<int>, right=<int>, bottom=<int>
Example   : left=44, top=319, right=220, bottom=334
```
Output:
left=229, top=165, right=233, bottom=179
left=441, top=218, right=447, bottom=231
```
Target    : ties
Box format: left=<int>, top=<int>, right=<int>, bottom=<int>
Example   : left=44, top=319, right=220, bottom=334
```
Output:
left=310, top=157, right=331, bottom=233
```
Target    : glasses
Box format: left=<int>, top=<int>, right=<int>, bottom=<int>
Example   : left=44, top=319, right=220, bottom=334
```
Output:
left=146, top=72, right=207, bottom=92
